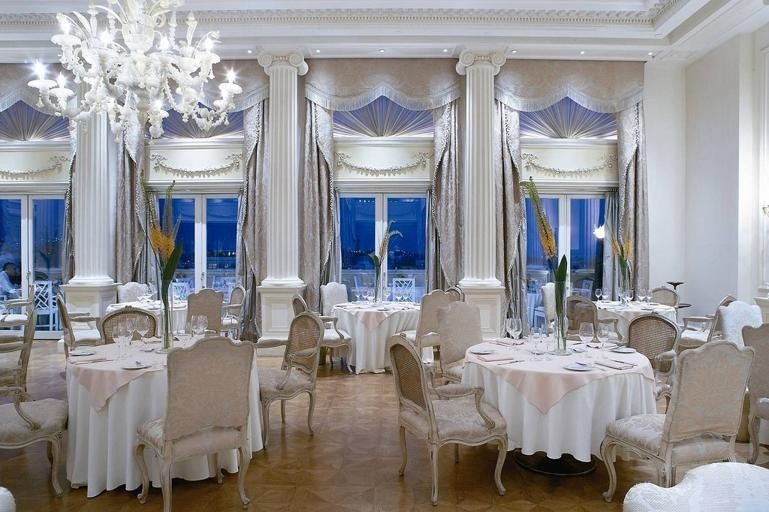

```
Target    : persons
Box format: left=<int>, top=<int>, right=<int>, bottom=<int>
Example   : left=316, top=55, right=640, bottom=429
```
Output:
left=0, top=261, right=22, bottom=330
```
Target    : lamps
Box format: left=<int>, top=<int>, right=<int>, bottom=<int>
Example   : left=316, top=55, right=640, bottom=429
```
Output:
left=28, top=0, right=242, bottom=138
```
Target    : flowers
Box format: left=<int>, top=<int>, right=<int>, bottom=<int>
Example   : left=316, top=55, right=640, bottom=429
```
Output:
left=522, top=176, right=568, bottom=350
left=606, top=223, right=631, bottom=302
left=137, top=171, right=182, bottom=345
left=368, top=221, right=402, bottom=302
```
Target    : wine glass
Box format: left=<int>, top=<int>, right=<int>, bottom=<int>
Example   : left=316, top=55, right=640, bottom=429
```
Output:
left=135, top=285, right=158, bottom=307
left=352, top=288, right=414, bottom=309
left=505, top=315, right=613, bottom=361
left=110, top=312, right=208, bottom=358
left=593, top=285, right=655, bottom=306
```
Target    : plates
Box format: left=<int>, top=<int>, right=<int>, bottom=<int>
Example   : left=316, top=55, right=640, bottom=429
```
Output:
left=615, top=346, right=638, bottom=355
left=121, top=363, right=152, bottom=372
left=564, top=363, right=591, bottom=372
left=472, top=347, right=494, bottom=356
left=68, top=349, right=96, bottom=358
